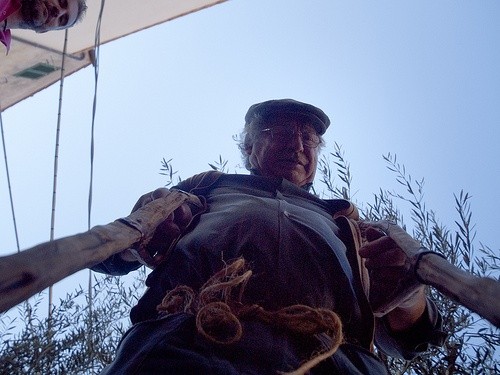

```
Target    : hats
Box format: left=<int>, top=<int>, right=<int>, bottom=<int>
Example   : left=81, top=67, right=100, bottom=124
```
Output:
left=245, top=98, right=330, bottom=135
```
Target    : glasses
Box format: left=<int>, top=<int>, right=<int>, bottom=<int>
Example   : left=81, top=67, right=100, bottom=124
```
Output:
left=259, top=126, right=322, bottom=148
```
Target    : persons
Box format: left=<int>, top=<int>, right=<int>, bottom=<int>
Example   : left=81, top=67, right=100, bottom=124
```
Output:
left=88, top=98, right=446, bottom=375
left=0, top=0, right=88, bottom=55
